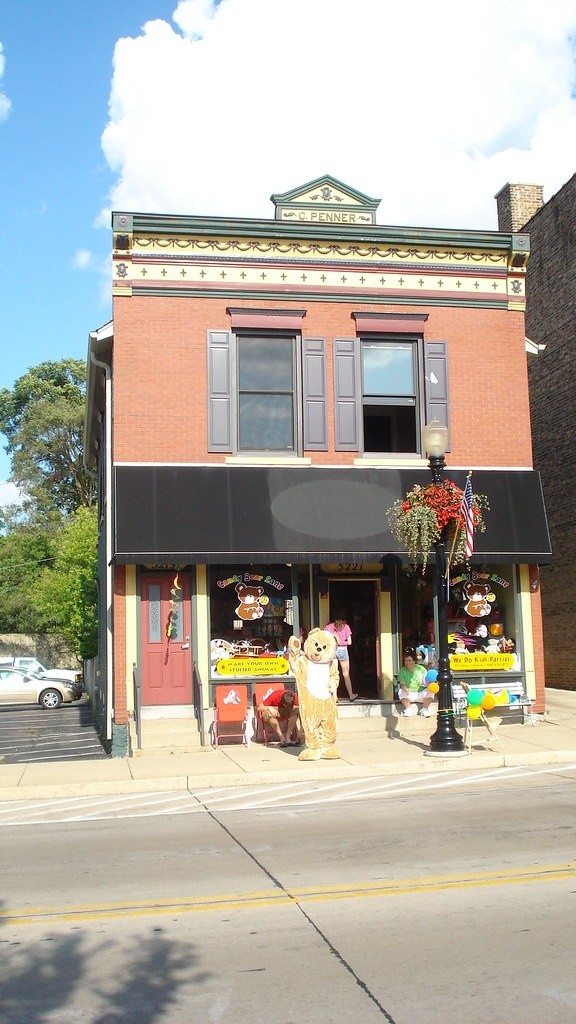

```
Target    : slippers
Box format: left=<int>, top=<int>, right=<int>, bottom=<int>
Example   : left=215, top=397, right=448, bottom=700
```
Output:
left=288, top=741, right=301, bottom=747
left=279, top=740, right=288, bottom=746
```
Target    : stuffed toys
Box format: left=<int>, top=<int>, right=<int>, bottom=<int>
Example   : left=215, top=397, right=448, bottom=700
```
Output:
left=288, top=626, right=340, bottom=761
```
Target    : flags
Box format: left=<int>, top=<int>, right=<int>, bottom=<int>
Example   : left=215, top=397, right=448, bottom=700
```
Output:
left=461, top=479, right=476, bottom=559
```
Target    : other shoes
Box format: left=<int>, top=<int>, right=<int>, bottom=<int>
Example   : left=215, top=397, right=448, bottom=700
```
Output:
left=350, top=694, right=359, bottom=701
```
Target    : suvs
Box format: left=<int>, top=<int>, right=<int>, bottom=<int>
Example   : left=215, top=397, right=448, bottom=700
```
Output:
left=0, top=657, right=84, bottom=689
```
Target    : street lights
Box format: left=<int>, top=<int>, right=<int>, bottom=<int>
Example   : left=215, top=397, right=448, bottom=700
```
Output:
left=421, top=417, right=468, bottom=756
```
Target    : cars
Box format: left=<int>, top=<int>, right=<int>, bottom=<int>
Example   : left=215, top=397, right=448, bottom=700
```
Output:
left=0, top=668, right=82, bottom=709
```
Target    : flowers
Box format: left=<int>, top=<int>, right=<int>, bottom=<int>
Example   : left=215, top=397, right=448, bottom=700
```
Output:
left=386, top=479, right=490, bottom=576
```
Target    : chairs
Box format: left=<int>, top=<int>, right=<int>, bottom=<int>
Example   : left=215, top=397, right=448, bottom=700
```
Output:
left=213, top=685, right=249, bottom=749
left=253, top=683, right=301, bottom=747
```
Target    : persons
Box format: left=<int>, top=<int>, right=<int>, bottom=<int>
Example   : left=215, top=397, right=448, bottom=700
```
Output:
left=257, top=689, right=301, bottom=748
left=396, top=652, right=435, bottom=718
left=323, top=613, right=359, bottom=703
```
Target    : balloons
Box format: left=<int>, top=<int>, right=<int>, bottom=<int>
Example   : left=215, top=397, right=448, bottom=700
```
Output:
left=424, top=676, right=431, bottom=686
left=467, top=706, right=482, bottom=719
left=427, top=682, right=440, bottom=694
left=426, top=669, right=438, bottom=683
left=480, top=694, right=495, bottom=710
left=467, top=688, right=483, bottom=706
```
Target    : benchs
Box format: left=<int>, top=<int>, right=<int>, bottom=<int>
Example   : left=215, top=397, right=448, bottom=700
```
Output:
left=451, top=682, right=535, bottom=732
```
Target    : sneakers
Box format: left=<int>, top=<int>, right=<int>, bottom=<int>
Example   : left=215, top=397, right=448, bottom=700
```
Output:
left=403, top=707, right=417, bottom=716
left=420, top=708, right=430, bottom=719
